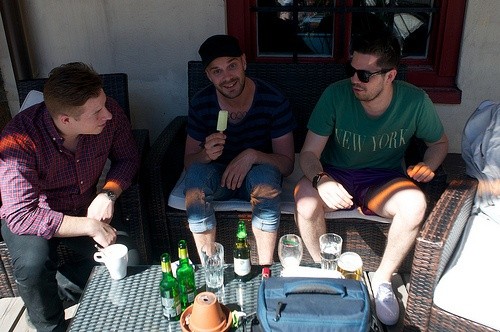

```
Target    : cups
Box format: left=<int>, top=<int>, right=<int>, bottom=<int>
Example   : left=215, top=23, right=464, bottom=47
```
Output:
left=319, top=233, right=343, bottom=270
left=278, top=234, right=303, bottom=268
left=336, top=252, right=362, bottom=280
left=200, top=242, right=225, bottom=289
left=93, top=244, right=129, bottom=280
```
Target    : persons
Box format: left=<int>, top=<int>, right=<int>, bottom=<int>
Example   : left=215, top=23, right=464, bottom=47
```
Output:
left=0, top=61, right=137, bottom=332
left=183, top=35, right=294, bottom=267
left=284, top=32, right=449, bottom=324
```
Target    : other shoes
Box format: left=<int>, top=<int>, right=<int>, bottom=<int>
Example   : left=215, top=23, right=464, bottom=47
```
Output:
left=25, top=309, right=36, bottom=330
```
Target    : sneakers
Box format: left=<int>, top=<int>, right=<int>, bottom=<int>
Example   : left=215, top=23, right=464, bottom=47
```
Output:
left=371, top=275, right=399, bottom=325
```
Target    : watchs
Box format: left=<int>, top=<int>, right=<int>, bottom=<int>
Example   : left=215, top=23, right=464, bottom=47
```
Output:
left=100, top=189, right=118, bottom=202
left=312, top=173, right=331, bottom=190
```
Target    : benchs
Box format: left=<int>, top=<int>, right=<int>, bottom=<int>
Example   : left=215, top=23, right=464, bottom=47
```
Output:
left=144, top=61, right=442, bottom=275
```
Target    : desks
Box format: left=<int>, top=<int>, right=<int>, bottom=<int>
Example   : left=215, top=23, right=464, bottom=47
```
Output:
left=68, top=264, right=379, bottom=332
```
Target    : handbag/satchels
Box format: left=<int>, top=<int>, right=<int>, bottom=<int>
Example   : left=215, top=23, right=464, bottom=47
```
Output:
left=252, top=277, right=370, bottom=332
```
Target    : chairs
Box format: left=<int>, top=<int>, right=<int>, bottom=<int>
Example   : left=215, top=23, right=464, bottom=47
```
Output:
left=401, top=100, right=500, bottom=332
left=0, top=73, right=147, bottom=298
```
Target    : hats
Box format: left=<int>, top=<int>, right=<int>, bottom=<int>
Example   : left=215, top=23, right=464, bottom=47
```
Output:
left=198, top=35, right=243, bottom=70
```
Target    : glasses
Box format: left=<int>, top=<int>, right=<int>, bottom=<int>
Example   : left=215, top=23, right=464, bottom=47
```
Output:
left=348, top=63, right=394, bottom=83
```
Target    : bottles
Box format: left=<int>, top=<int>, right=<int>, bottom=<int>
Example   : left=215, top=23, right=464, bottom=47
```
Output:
left=176, top=240, right=196, bottom=312
left=159, top=253, right=182, bottom=321
left=232, top=221, right=253, bottom=283
